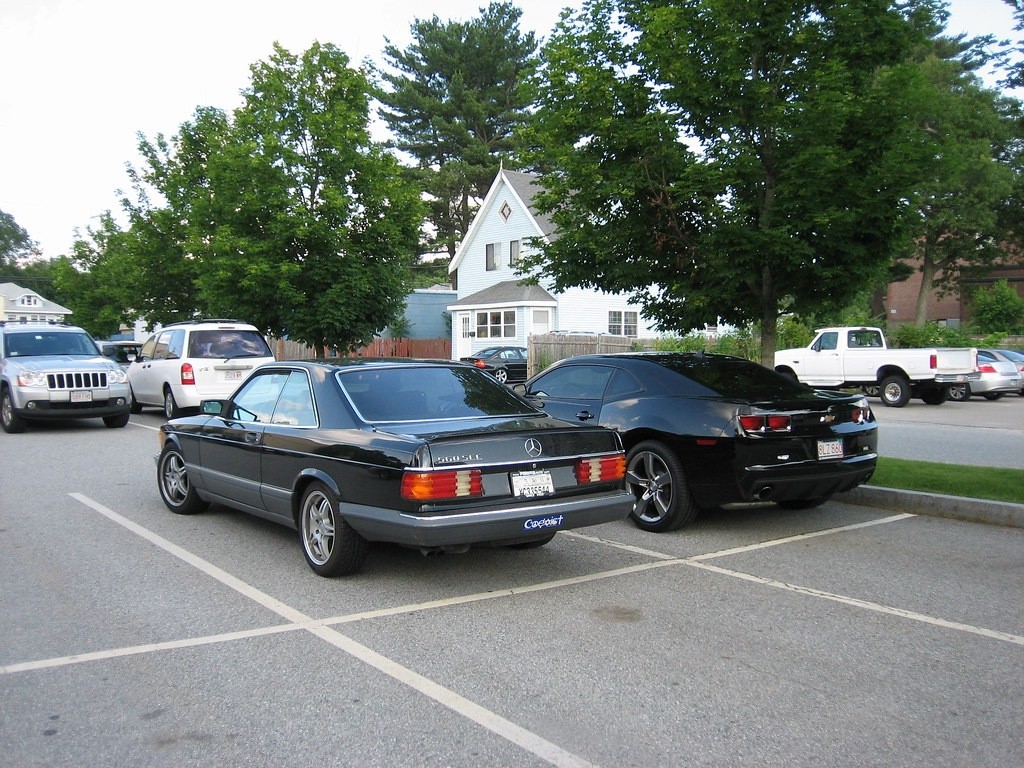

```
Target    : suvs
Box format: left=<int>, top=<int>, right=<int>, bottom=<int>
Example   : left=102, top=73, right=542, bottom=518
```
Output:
left=0, top=320, right=133, bottom=434
left=860, top=348, right=1022, bottom=402
left=127, top=319, right=277, bottom=420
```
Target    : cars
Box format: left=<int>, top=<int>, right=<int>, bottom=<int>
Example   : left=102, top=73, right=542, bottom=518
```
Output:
left=152, top=356, right=636, bottom=578
left=516, top=352, right=879, bottom=533
left=460, top=346, right=528, bottom=384
left=93, top=340, right=146, bottom=374
left=996, top=350, right=1024, bottom=398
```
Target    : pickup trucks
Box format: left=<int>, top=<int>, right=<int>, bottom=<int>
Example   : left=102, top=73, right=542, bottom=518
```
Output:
left=773, top=326, right=981, bottom=407
